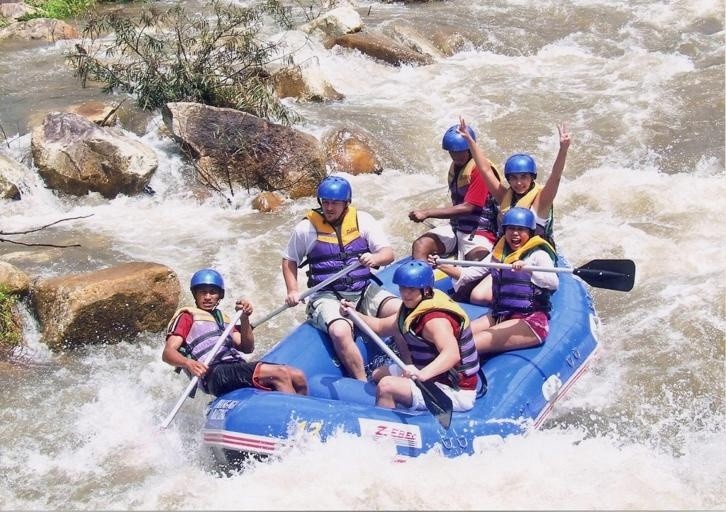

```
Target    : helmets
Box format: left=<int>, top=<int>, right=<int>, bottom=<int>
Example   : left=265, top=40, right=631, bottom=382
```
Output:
left=393, top=260, right=435, bottom=291
left=442, top=124, right=476, bottom=152
left=317, top=175, right=352, bottom=202
left=190, top=268, right=224, bottom=290
left=504, top=153, right=537, bottom=180
left=502, top=207, right=536, bottom=235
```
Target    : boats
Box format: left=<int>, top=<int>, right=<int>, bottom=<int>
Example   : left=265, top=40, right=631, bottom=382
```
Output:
left=201, top=243, right=602, bottom=474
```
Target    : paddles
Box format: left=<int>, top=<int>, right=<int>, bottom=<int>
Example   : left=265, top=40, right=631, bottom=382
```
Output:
left=342, top=297, right=453, bottom=429
left=235, top=253, right=379, bottom=333
left=431, top=250, right=635, bottom=292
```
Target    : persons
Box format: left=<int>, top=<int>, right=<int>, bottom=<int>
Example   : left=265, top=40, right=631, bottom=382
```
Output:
left=163, top=270, right=308, bottom=396
left=282, top=178, right=405, bottom=382
left=408, top=125, right=501, bottom=261
left=428, top=207, right=560, bottom=355
left=340, top=260, right=480, bottom=412
left=456, top=115, right=572, bottom=305
